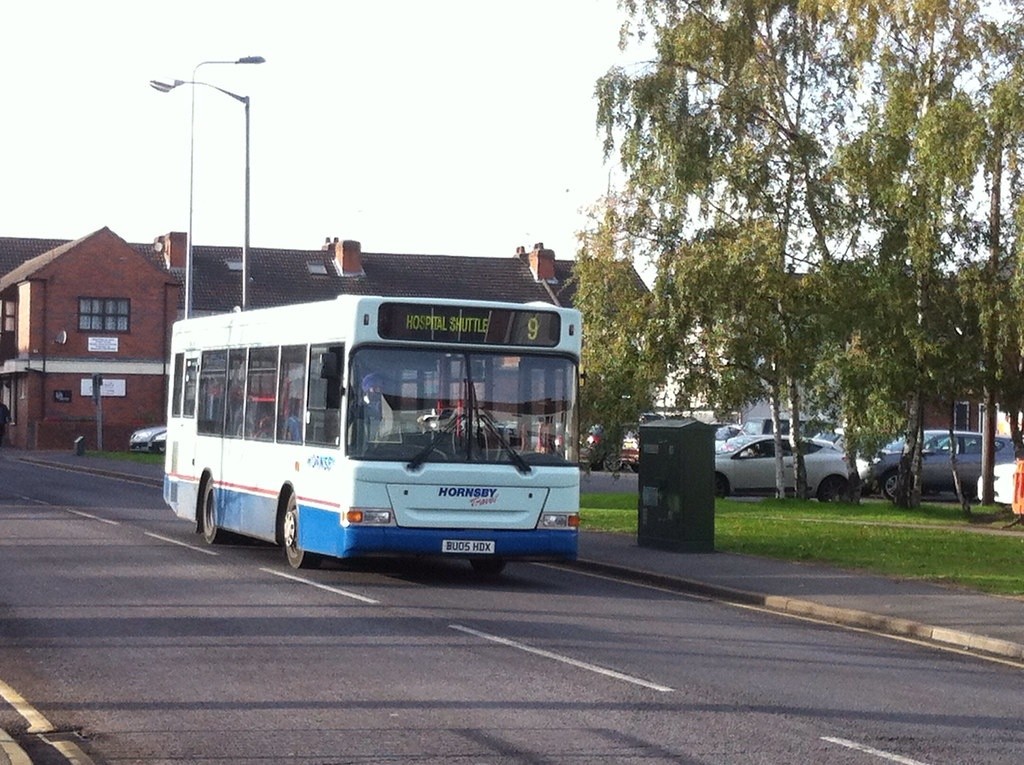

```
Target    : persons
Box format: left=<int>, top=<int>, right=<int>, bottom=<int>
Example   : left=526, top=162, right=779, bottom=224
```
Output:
left=0, top=401, right=12, bottom=447
left=247, top=411, right=300, bottom=441
left=352, top=374, right=396, bottom=449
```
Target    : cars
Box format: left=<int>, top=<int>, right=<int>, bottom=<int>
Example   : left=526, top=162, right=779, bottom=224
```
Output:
left=604, top=410, right=869, bottom=474
left=869, top=428, right=1014, bottom=501
left=129, top=425, right=168, bottom=454
left=714, top=432, right=871, bottom=504
left=975, top=443, right=1024, bottom=505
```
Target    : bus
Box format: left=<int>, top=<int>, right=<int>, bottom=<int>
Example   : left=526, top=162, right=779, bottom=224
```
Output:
left=159, top=298, right=583, bottom=570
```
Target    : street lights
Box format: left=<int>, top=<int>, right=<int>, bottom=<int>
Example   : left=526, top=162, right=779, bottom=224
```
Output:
left=149, top=78, right=255, bottom=310
left=183, top=56, right=269, bottom=320
